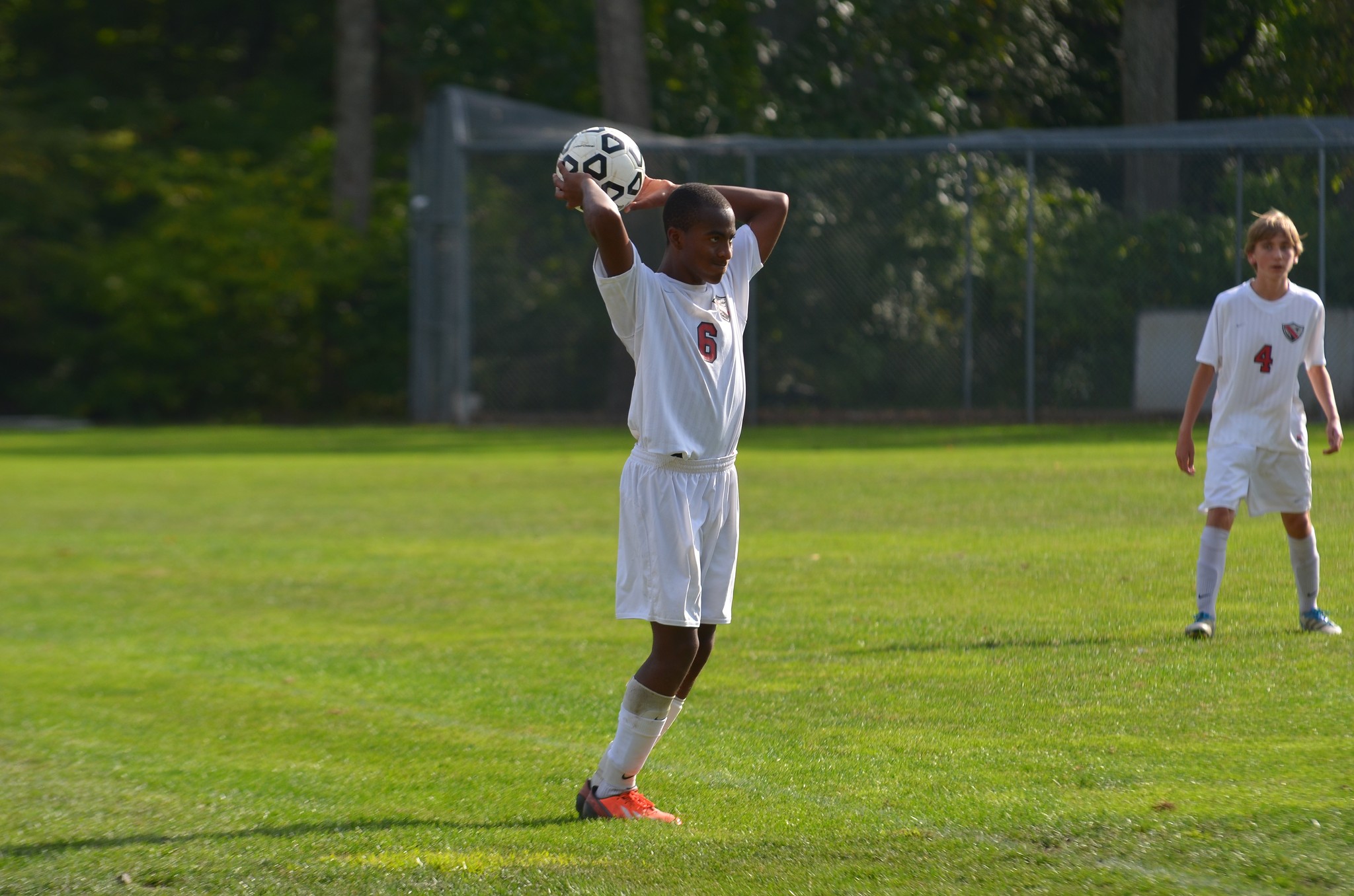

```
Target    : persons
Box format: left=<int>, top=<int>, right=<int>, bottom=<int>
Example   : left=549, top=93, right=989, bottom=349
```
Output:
left=1176, top=207, right=1347, bottom=638
left=553, top=163, right=788, bottom=824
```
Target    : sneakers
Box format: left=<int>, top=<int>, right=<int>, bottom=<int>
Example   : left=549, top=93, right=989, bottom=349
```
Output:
left=1185, top=611, right=1216, bottom=639
left=1300, top=609, right=1343, bottom=635
left=575, top=778, right=681, bottom=824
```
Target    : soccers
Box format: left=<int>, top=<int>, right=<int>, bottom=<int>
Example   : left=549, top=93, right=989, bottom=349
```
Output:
left=559, top=125, right=647, bottom=213
